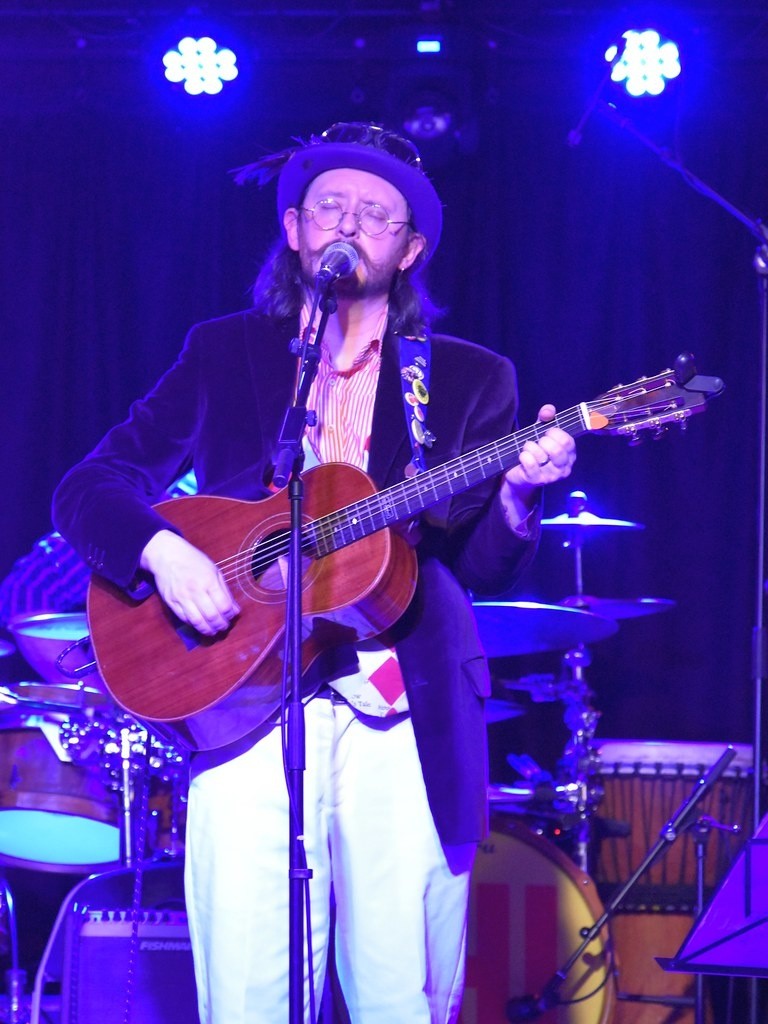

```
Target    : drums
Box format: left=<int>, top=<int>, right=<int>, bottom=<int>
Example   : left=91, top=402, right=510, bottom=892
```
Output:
left=449, top=812, right=614, bottom=1024
left=0, top=680, right=152, bottom=876
left=563, top=736, right=757, bottom=1024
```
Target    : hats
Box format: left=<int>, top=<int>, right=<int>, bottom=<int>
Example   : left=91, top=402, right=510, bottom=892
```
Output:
left=228, top=123, right=442, bottom=281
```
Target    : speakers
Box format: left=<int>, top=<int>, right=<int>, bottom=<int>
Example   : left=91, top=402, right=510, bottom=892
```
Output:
left=59, top=903, right=203, bottom=1024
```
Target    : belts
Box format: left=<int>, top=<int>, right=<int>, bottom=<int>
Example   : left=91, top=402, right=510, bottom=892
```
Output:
left=313, top=681, right=348, bottom=704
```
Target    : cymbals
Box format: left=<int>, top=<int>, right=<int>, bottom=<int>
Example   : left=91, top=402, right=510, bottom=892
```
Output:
left=564, top=597, right=676, bottom=620
left=486, top=699, right=530, bottom=724
left=13, top=610, right=88, bottom=645
left=538, top=513, right=645, bottom=532
left=474, top=598, right=620, bottom=662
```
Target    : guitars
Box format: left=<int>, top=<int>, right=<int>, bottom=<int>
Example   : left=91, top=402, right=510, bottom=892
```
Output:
left=84, top=352, right=729, bottom=759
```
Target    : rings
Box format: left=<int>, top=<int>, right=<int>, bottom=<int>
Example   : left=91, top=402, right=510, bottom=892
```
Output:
left=539, top=455, right=551, bottom=467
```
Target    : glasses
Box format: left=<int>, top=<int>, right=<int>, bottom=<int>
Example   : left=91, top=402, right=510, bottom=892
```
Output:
left=300, top=198, right=412, bottom=235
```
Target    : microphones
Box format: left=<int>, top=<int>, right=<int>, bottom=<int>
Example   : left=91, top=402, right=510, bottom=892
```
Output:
left=317, top=242, right=359, bottom=282
left=506, top=993, right=559, bottom=1024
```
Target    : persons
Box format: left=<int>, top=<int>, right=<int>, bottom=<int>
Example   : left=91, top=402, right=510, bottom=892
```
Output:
left=50, top=124, right=576, bottom=1024
left=0, top=470, right=198, bottom=698
